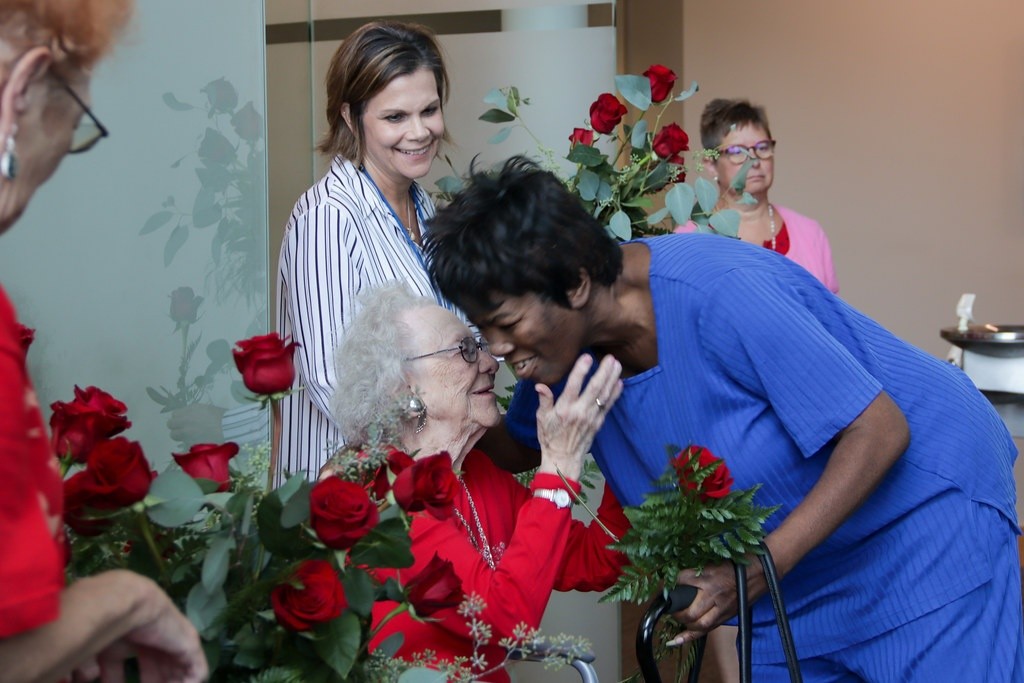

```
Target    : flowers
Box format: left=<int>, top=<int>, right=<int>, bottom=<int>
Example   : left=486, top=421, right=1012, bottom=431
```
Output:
left=139, top=78, right=267, bottom=337
left=437, top=65, right=758, bottom=244
left=558, top=444, right=784, bottom=683
left=147, top=288, right=230, bottom=411
left=48, top=332, right=592, bottom=683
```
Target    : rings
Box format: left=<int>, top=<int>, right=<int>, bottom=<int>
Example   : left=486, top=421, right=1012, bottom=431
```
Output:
left=595, top=397, right=606, bottom=409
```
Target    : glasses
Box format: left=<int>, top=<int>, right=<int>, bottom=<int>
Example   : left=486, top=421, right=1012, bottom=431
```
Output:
left=401, top=337, right=493, bottom=364
left=49, top=69, right=108, bottom=153
left=713, top=140, right=776, bottom=164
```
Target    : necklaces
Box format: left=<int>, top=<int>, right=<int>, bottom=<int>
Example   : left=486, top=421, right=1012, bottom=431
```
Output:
left=768, top=204, right=776, bottom=251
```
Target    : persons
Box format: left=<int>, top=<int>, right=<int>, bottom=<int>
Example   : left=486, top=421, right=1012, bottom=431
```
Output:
left=275, top=19, right=484, bottom=489
left=421, top=154, right=1023, bottom=683
left=320, top=278, right=632, bottom=683
left=669, top=99, right=838, bottom=298
left=0, top=0, right=209, bottom=683
